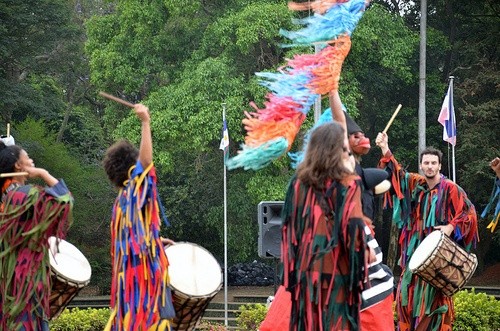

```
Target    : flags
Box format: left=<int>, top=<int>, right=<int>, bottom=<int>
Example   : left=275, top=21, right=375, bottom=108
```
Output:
left=437, top=78, right=457, bottom=146
left=218, top=107, right=230, bottom=164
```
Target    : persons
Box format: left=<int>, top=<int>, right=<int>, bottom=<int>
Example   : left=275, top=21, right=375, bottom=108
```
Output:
left=258, top=90, right=500, bottom=331
left=0, top=134, right=76, bottom=331
left=103, top=104, right=177, bottom=331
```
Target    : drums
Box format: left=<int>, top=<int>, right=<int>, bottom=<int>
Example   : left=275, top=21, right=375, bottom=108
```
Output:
left=408, top=229, right=478, bottom=297
left=46, top=236, right=92, bottom=321
left=162, top=241, right=224, bottom=331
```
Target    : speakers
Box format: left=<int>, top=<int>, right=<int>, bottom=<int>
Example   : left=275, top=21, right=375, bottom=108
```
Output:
left=257, top=201, right=285, bottom=259
